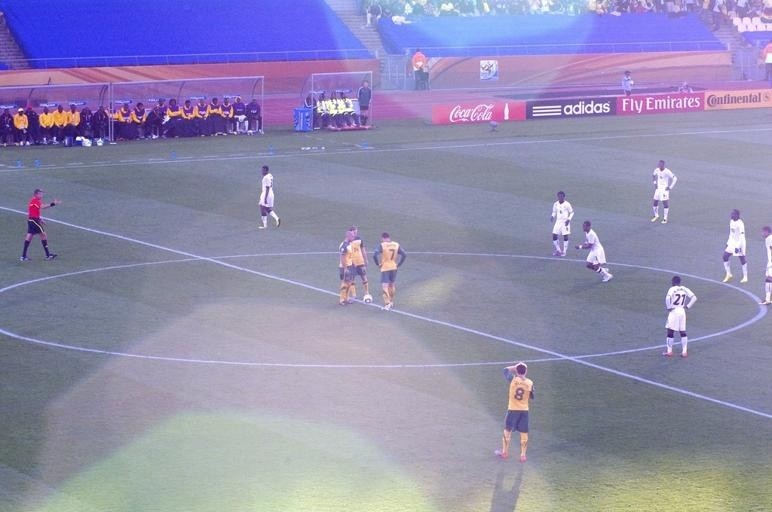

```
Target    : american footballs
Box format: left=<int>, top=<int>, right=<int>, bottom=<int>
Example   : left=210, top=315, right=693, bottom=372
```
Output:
left=413, top=51, right=426, bottom=69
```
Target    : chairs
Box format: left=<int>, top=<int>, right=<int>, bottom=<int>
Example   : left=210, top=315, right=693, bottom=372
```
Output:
left=320, top=100, right=361, bottom=128
left=733, top=16, right=772, bottom=32
left=143, top=108, right=258, bottom=138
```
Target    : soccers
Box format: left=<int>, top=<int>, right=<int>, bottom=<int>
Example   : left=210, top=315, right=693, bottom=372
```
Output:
left=364, top=294, right=373, bottom=304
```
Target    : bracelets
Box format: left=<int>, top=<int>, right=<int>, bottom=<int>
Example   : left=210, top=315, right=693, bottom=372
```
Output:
left=50, top=202, right=56, bottom=207
left=579, top=244, right=582, bottom=249
left=653, top=180, right=656, bottom=185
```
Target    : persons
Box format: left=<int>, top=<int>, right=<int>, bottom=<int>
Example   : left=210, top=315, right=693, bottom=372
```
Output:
left=361, top=0, right=771, bottom=33
left=575, top=220, right=613, bottom=282
left=20, top=189, right=63, bottom=261
left=621, top=71, right=633, bottom=96
left=305, top=81, right=371, bottom=131
left=339, top=227, right=406, bottom=311
left=411, top=48, right=428, bottom=91
left=762, top=40, right=772, bottom=81
left=721, top=209, right=747, bottom=284
left=494, top=362, right=535, bottom=462
left=661, top=276, right=697, bottom=358
left=651, top=160, right=678, bottom=224
left=757, top=226, right=772, bottom=305
left=550, top=192, right=574, bottom=256
left=258, top=165, right=280, bottom=229
left=0, top=95, right=263, bottom=147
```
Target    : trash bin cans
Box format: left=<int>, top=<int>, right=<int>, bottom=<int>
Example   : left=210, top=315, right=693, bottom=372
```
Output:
left=293, top=108, right=312, bottom=132
